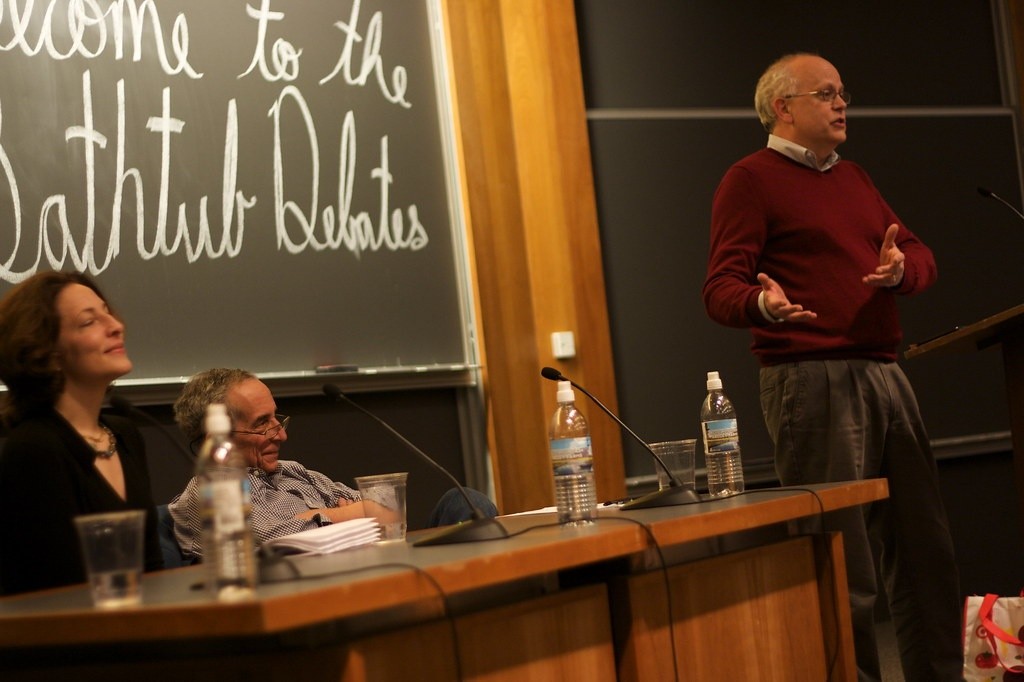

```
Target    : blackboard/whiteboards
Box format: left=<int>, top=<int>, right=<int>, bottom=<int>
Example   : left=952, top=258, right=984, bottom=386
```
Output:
left=572, top=0, right=1024, bottom=503
left=0, top=1, right=489, bottom=410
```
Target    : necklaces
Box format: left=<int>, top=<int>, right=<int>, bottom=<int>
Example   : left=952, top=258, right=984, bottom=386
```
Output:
left=93, top=421, right=116, bottom=457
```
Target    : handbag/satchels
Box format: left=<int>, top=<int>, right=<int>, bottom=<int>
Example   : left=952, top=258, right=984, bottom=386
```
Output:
left=961, top=591, right=1024, bottom=682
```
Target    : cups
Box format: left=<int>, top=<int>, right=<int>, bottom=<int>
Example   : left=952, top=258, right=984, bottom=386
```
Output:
left=650, top=439, right=696, bottom=494
left=74, top=510, right=146, bottom=610
left=354, top=472, right=408, bottom=545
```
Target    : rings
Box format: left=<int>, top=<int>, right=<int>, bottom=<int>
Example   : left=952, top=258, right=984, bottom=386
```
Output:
left=893, top=275, right=896, bottom=283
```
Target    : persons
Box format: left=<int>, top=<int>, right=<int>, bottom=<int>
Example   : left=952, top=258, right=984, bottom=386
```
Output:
left=702, top=53, right=964, bottom=682
left=167, top=369, right=498, bottom=563
left=0, top=271, right=163, bottom=596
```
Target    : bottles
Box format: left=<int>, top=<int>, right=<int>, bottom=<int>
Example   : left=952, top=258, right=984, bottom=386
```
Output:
left=194, top=404, right=258, bottom=601
left=549, top=380, right=599, bottom=528
left=701, top=372, right=745, bottom=499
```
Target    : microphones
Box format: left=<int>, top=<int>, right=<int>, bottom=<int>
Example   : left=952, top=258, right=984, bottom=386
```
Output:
left=541, top=367, right=700, bottom=511
left=108, top=395, right=283, bottom=566
left=976, top=185, right=1024, bottom=221
left=323, top=379, right=509, bottom=546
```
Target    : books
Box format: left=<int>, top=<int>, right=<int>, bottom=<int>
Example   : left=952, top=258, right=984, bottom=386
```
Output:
left=257, top=515, right=409, bottom=578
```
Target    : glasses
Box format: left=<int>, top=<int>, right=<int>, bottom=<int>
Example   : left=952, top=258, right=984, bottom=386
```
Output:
left=233, top=413, right=291, bottom=440
left=785, top=89, right=852, bottom=105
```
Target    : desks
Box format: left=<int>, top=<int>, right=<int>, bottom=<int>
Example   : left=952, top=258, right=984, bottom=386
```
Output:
left=0, top=475, right=889, bottom=682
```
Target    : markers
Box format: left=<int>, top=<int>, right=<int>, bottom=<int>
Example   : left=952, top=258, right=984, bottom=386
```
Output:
left=316, top=364, right=359, bottom=373
